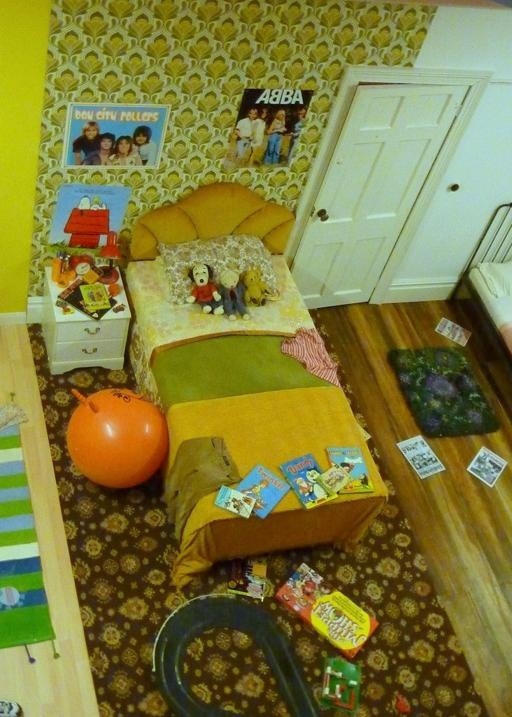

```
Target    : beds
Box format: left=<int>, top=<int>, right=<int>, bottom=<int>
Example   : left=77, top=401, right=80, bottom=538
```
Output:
left=445, top=198, right=512, bottom=428
left=125, top=180, right=392, bottom=592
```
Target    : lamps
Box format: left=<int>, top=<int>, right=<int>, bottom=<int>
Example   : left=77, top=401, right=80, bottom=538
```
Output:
left=97, top=232, right=120, bottom=284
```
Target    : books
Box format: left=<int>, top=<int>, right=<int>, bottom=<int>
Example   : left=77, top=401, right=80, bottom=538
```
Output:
left=57, top=277, right=118, bottom=322
left=80, top=284, right=111, bottom=310
left=278, top=453, right=338, bottom=511
left=235, top=462, right=291, bottom=520
left=465, top=446, right=508, bottom=488
left=396, top=434, right=446, bottom=479
left=214, top=484, right=257, bottom=521
left=315, top=462, right=350, bottom=497
left=434, top=317, right=472, bottom=347
left=325, top=447, right=374, bottom=493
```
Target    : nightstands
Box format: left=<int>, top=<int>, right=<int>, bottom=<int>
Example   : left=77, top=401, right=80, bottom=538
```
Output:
left=46, top=264, right=131, bottom=375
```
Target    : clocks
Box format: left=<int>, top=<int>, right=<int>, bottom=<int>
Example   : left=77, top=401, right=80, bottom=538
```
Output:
left=69, top=254, right=92, bottom=275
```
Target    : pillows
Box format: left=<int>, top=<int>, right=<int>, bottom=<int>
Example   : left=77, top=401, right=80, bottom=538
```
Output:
left=155, top=233, right=280, bottom=304
left=475, top=256, right=510, bottom=299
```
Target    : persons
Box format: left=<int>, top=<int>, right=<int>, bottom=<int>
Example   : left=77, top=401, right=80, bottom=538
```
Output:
left=247, top=106, right=272, bottom=166
left=287, top=105, right=307, bottom=158
left=235, top=107, right=257, bottom=166
left=84, top=131, right=116, bottom=165
left=72, top=122, right=105, bottom=164
left=263, top=108, right=287, bottom=165
left=107, top=136, right=142, bottom=165
left=133, top=125, right=156, bottom=165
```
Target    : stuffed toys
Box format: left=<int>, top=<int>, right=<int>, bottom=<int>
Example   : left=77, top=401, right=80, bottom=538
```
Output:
left=185, top=263, right=225, bottom=314
left=218, top=269, right=250, bottom=321
left=240, top=264, right=282, bottom=307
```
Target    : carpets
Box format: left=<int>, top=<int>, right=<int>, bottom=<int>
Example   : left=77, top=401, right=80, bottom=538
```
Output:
left=1, top=389, right=61, bottom=667
left=27, top=322, right=491, bottom=716
left=387, top=345, right=499, bottom=441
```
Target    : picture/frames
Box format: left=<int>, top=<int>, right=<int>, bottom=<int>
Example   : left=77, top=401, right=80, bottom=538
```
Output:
left=60, top=102, right=172, bottom=171
